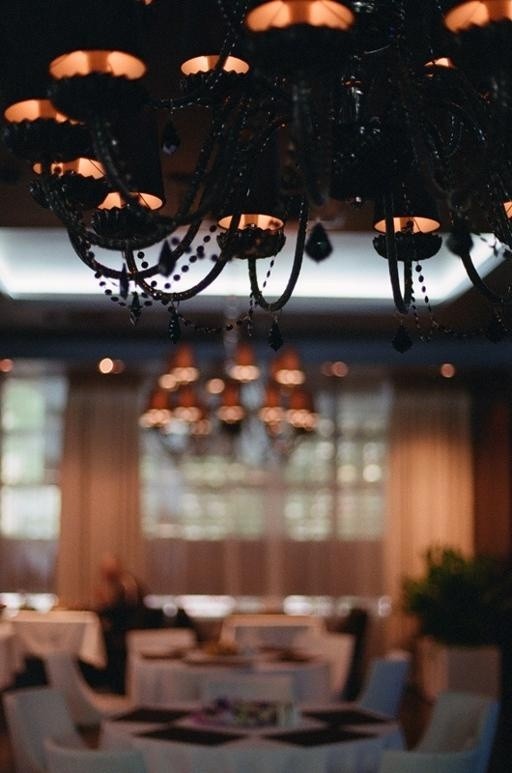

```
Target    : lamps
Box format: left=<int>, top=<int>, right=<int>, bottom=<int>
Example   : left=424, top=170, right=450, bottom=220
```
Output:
left=143, top=320, right=323, bottom=496
left=1, top=1, right=509, bottom=350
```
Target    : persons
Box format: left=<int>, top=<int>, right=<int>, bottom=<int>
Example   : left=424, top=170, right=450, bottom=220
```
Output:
left=0, top=555, right=149, bottom=696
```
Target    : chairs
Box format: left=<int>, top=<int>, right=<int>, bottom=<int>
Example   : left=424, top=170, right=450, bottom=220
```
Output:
left=2, top=602, right=510, bottom=770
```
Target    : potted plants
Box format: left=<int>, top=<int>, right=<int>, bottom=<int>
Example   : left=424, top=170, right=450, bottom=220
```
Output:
left=398, top=540, right=511, bottom=704
